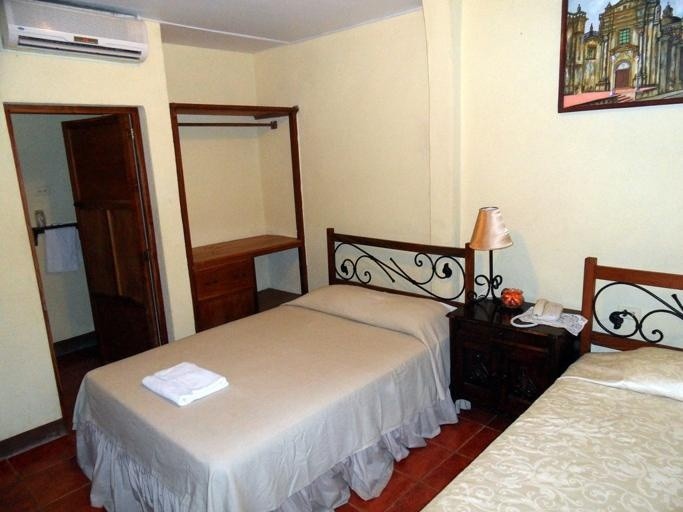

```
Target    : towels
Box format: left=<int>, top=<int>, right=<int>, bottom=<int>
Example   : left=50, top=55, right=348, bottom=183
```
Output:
left=44, top=228, right=83, bottom=276
left=140, top=361, right=230, bottom=406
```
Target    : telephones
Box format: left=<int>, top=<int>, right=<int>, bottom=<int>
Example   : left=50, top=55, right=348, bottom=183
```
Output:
left=533, top=299, right=564, bottom=322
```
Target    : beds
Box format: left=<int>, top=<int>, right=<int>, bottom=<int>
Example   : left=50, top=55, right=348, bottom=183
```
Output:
left=420, top=257, right=683, bottom=512
left=71, top=228, right=475, bottom=511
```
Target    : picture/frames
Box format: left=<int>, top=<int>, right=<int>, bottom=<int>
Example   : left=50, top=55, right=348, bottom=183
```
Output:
left=558, top=0, right=683, bottom=113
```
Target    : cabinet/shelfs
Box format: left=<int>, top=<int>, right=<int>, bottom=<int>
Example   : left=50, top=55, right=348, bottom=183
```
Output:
left=169, top=103, right=308, bottom=333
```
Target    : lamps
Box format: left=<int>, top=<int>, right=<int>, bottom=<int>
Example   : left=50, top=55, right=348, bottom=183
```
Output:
left=468, top=207, right=512, bottom=307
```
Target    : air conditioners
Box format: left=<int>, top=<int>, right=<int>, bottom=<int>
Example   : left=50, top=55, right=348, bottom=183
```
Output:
left=0, top=0, right=148, bottom=64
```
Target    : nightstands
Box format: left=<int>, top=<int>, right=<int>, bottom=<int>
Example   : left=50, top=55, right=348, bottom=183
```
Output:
left=446, top=299, right=584, bottom=421
